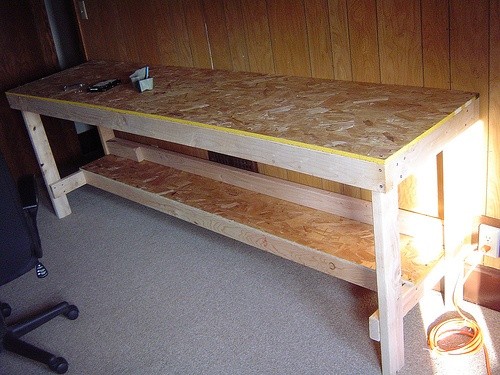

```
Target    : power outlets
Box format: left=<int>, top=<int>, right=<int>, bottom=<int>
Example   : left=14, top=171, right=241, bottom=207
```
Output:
left=478, top=224, right=500, bottom=258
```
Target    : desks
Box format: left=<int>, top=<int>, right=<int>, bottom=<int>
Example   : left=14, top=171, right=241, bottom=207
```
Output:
left=5, top=58, right=481, bottom=375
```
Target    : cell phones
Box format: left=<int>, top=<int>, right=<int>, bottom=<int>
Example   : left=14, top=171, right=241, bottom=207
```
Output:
left=88, top=79, right=121, bottom=92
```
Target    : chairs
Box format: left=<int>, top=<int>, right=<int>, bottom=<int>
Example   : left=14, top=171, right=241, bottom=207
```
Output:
left=0, top=151, right=79, bottom=375
